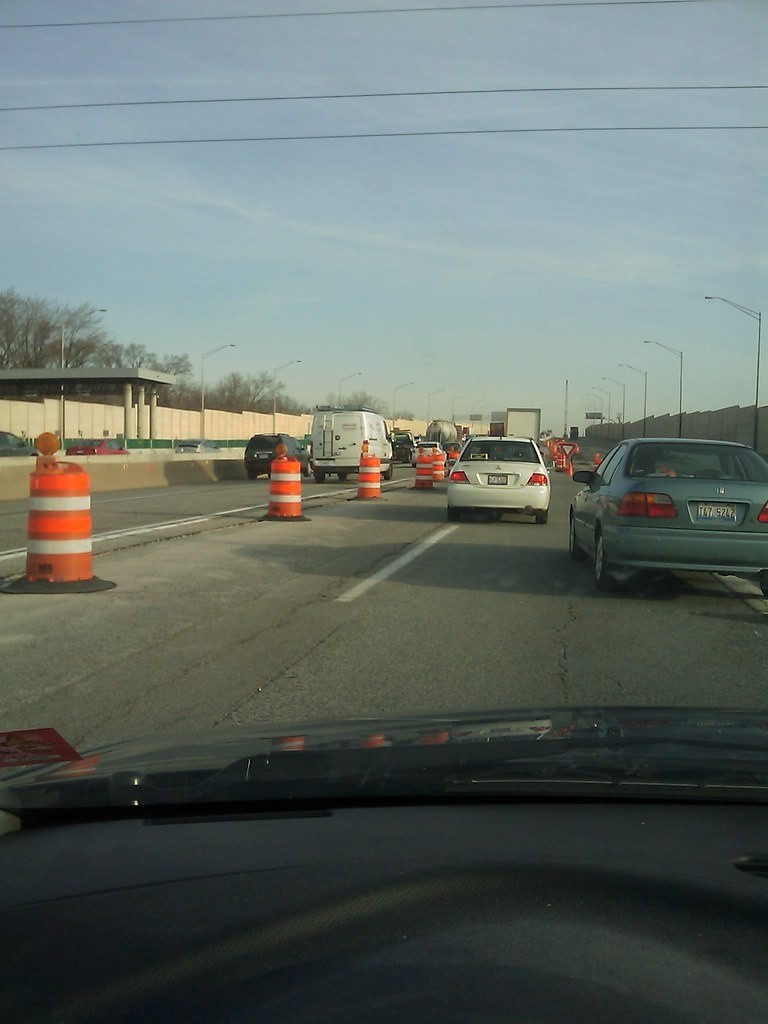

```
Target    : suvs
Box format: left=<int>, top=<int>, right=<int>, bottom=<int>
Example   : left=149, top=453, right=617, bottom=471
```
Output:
left=244, top=433, right=311, bottom=480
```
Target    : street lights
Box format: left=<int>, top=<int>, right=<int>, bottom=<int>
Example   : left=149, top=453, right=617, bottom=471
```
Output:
left=578, top=387, right=610, bottom=439
left=705, top=296, right=761, bottom=451
left=200, top=344, right=235, bottom=442
left=427, top=389, right=445, bottom=427
left=273, top=360, right=302, bottom=435
left=602, top=378, right=625, bottom=440
left=452, top=394, right=494, bottom=422
left=393, top=382, right=414, bottom=431
left=644, top=340, right=682, bottom=437
left=61, top=309, right=107, bottom=451
left=339, top=373, right=362, bottom=406
left=619, top=364, right=648, bottom=437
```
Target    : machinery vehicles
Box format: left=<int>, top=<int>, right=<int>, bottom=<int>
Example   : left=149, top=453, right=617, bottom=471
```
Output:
left=426, top=420, right=470, bottom=442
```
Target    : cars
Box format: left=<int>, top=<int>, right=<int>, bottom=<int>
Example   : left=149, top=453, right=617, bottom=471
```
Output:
left=569, top=437, right=768, bottom=599
left=65, top=439, right=131, bottom=455
left=389, top=432, right=462, bottom=468
left=0, top=704, right=768, bottom=1024
left=447, top=435, right=556, bottom=524
left=175, top=439, right=223, bottom=453
left=0, top=431, right=39, bottom=456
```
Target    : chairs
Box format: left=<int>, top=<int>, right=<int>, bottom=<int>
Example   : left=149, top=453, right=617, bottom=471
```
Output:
left=695, top=469, right=724, bottom=481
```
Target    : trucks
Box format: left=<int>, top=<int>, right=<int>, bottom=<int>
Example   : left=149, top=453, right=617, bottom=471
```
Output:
left=310, top=405, right=395, bottom=483
